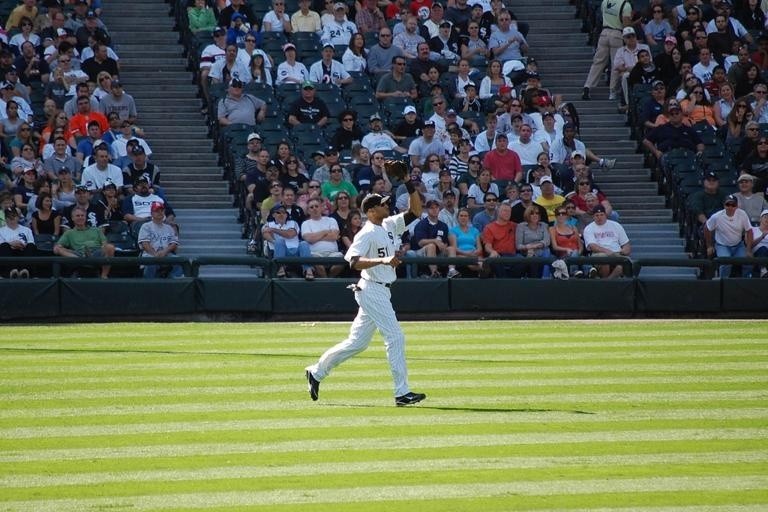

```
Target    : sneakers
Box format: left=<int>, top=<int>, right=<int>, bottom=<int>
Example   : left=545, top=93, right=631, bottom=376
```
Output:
left=446, top=268, right=461, bottom=280
left=306, top=369, right=320, bottom=403
left=571, top=269, right=584, bottom=278
left=588, top=268, right=599, bottom=278
left=608, top=93, right=618, bottom=100
left=430, top=270, right=443, bottom=279
left=394, top=392, right=425, bottom=406
left=601, top=156, right=617, bottom=173
left=582, top=85, right=591, bottom=101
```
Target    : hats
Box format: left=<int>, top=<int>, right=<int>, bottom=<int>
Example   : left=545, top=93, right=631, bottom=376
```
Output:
left=311, top=144, right=339, bottom=161
left=533, top=163, right=553, bottom=186
left=362, top=192, right=392, bottom=213
left=247, top=133, right=264, bottom=142
left=701, top=170, right=768, bottom=218
left=422, top=120, right=435, bottom=129
left=571, top=150, right=584, bottom=159
left=212, top=1, right=345, bottom=64
left=301, top=79, right=315, bottom=89
left=369, top=114, right=382, bottom=122
left=228, top=78, right=243, bottom=87
left=403, top=105, right=417, bottom=115
left=0, top=6, right=165, bottom=220
left=537, top=96, right=578, bottom=136
left=582, top=192, right=606, bottom=213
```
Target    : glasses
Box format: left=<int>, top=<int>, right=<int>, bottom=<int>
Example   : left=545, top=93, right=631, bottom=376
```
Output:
left=578, top=181, right=590, bottom=187
left=555, top=206, right=576, bottom=216
left=342, top=117, right=354, bottom=122
left=651, top=10, right=768, bottom=150
left=373, top=156, right=385, bottom=160
left=428, top=158, right=533, bottom=205
left=265, top=160, right=350, bottom=218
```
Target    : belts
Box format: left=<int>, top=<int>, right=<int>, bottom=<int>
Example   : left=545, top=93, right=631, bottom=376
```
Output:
left=376, top=282, right=393, bottom=288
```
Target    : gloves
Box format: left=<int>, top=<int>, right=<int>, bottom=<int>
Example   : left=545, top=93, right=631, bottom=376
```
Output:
left=346, top=283, right=362, bottom=291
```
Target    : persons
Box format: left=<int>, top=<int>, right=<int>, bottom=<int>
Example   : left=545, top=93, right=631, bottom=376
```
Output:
left=304, top=159, right=427, bottom=407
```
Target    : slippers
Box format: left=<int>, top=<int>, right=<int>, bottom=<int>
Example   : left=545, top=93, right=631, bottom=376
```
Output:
left=305, top=272, right=315, bottom=280
left=275, top=269, right=288, bottom=278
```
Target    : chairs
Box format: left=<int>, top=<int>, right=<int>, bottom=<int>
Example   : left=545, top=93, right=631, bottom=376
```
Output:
left=178, top=1, right=381, bottom=83
left=97, top=218, right=156, bottom=257
left=26, top=81, right=74, bottom=135
left=209, top=83, right=486, bottom=258
left=35, top=232, right=58, bottom=252
left=623, top=83, right=764, bottom=257
left=579, top=1, right=652, bottom=46
